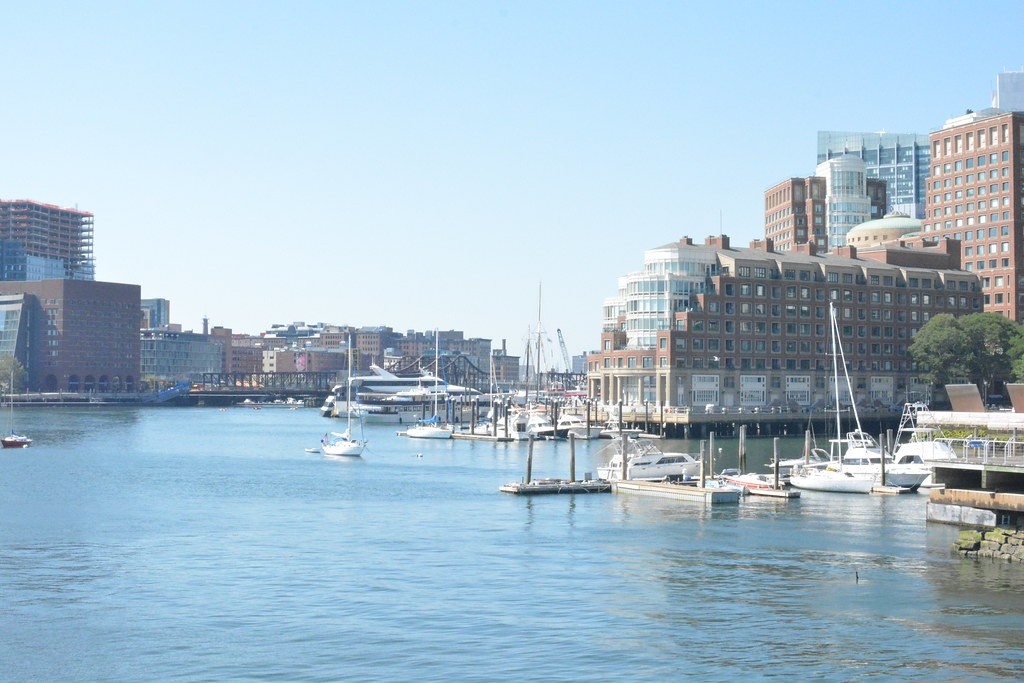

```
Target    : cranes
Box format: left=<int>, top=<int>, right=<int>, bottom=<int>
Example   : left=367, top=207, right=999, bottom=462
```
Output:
left=557, top=330, right=572, bottom=371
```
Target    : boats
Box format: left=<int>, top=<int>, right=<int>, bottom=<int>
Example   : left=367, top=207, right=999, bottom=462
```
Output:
left=597, top=440, right=707, bottom=480
left=492, top=397, right=643, bottom=439
left=764, top=401, right=958, bottom=476
left=239, top=397, right=305, bottom=410
left=320, top=364, right=500, bottom=424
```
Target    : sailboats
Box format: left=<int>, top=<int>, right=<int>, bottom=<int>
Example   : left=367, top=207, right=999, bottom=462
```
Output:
left=407, top=328, right=451, bottom=438
left=321, top=333, right=365, bottom=456
left=789, top=302, right=933, bottom=493
left=1, top=371, right=33, bottom=448
